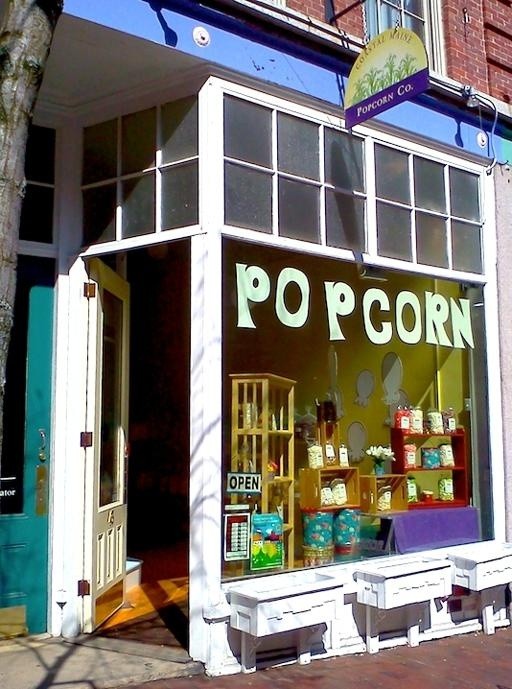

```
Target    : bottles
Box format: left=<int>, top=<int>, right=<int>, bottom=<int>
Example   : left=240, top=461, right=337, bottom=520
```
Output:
left=437, top=472, right=455, bottom=502
left=438, top=441, right=456, bottom=469
left=403, top=441, right=418, bottom=470
left=407, top=477, right=418, bottom=503
left=395, top=404, right=457, bottom=435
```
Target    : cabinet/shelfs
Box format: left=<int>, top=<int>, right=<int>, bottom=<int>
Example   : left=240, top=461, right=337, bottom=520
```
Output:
left=231, top=373, right=475, bottom=577
